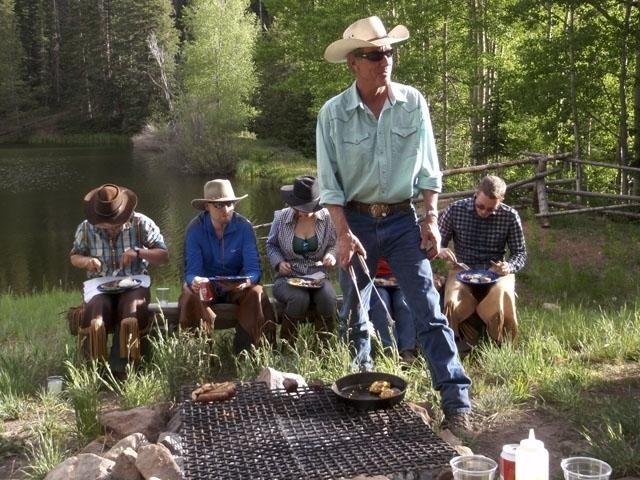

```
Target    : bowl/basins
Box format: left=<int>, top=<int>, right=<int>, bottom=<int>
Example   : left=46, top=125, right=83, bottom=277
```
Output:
left=331, top=372, right=408, bottom=407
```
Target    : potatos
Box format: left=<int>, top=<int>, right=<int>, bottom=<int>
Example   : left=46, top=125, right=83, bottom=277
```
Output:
left=370, top=381, right=401, bottom=399
left=310, top=379, right=324, bottom=391
left=283, top=379, right=297, bottom=392
left=193, top=382, right=236, bottom=401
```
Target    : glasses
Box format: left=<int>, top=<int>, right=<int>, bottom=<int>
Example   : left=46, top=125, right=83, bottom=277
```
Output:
left=209, top=202, right=235, bottom=210
left=352, top=48, right=396, bottom=63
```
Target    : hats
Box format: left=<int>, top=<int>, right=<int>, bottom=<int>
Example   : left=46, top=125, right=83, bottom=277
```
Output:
left=82, top=182, right=139, bottom=233
left=279, top=174, right=327, bottom=213
left=189, top=178, right=250, bottom=212
left=322, top=15, right=411, bottom=65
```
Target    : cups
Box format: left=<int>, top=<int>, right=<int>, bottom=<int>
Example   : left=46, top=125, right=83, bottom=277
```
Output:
left=449, top=455, right=498, bottom=480
left=156, top=287, right=170, bottom=305
left=561, top=456, right=613, bottom=480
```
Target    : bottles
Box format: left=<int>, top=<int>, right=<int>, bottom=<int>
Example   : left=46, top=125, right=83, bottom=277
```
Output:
left=47, top=376, right=62, bottom=393
left=515, top=428, right=550, bottom=480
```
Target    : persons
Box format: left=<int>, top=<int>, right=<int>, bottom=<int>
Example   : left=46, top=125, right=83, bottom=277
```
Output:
left=367, top=256, right=423, bottom=370
left=265, top=174, right=338, bottom=357
left=176, top=181, right=277, bottom=357
left=437, top=174, right=528, bottom=348
left=68, top=182, right=170, bottom=381
left=315, top=14, right=472, bottom=434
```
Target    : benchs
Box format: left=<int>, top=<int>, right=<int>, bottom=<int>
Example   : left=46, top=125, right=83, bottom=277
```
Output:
left=67, top=297, right=344, bottom=335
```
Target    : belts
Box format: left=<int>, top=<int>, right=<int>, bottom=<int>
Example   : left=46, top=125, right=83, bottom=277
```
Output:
left=343, top=196, right=413, bottom=220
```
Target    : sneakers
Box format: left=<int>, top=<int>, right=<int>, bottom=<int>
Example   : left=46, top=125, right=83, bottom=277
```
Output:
left=438, top=413, right=473, bottom=440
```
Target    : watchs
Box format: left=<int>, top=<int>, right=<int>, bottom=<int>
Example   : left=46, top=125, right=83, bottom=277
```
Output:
left=424, top=208, right=439, bottom=220
left=132, top=244, right=139, bottom=259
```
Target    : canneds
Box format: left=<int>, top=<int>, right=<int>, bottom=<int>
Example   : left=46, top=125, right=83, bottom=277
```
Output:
left=199, top=278, right=213, bottom=301
left=499, top=444, right=519, bottom=479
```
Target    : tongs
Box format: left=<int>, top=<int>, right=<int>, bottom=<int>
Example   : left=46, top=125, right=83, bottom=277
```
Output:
left=349, top=251, right=397, bottom=328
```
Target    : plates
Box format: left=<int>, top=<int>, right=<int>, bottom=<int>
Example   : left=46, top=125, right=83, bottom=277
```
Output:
left=209, top=276, right=251, bottom=285
left=287, top=277, right=325, bottom=290
left=455, top=269, right=501, bottom=285
left=96, top=279, right=143, bottom=293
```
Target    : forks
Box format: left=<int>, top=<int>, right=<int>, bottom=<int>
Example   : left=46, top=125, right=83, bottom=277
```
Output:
left=112, top=267, right=125, bottom=280
